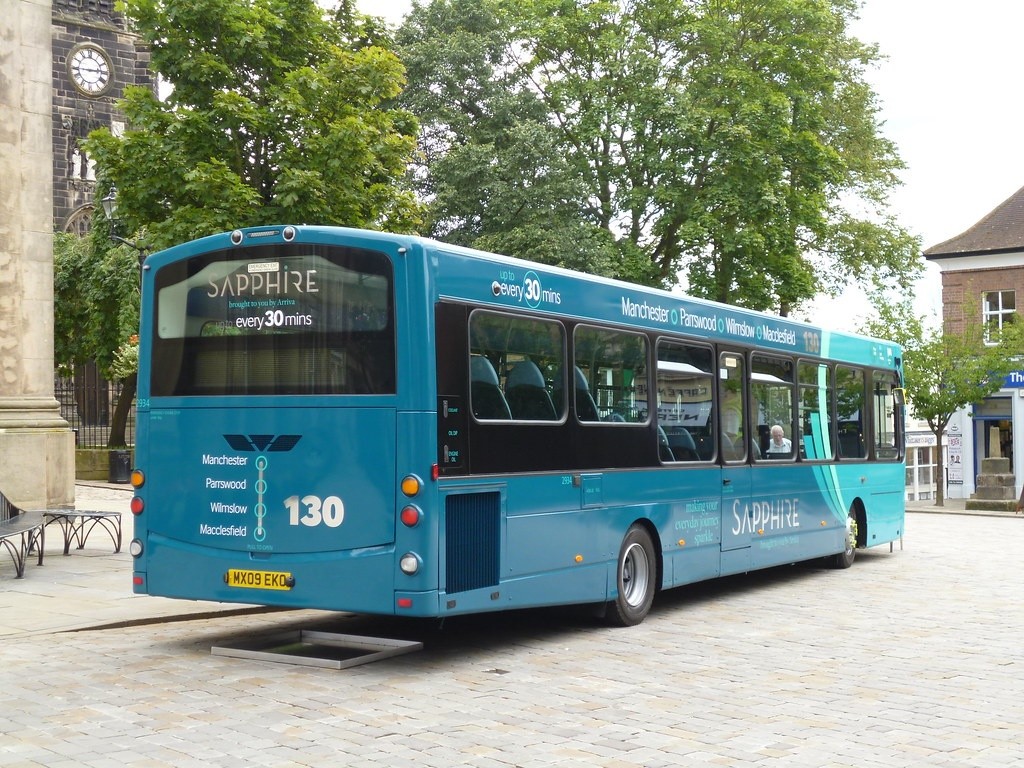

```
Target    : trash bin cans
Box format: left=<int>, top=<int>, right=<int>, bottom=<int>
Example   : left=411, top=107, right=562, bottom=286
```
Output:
left=107, top=451, right=132, bottom=483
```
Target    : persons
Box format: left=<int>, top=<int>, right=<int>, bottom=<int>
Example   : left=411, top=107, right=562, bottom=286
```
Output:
left=766, top=425, right=792, bottom=453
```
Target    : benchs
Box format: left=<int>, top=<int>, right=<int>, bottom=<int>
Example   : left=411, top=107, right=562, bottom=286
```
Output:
left=0, top=490, right=123, bottom=579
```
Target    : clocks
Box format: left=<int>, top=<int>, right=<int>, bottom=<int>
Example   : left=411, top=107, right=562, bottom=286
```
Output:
left=65, top=41, right=117, bottom=98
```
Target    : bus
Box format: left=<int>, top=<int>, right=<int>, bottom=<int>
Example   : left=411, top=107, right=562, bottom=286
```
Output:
left=130, top=224, right=908, bottom=627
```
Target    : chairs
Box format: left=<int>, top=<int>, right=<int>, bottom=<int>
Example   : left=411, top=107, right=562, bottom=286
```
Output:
left=505, top=361, right=558, bottom=420
left=702, top=432, right=738, bottom=461
left=667, top=428, right=701, bottom=461
left=641, top=419, right=675, bottom=461
left=601, top=413, right=626, bottom=422
left=470, top=356, right=512, bottom=420
left=733, top=437, right=763, bottom=461
left=552, top=363, right=601, bottom=422
